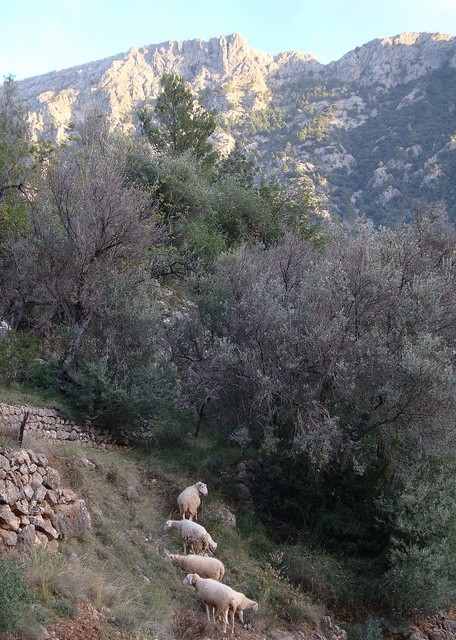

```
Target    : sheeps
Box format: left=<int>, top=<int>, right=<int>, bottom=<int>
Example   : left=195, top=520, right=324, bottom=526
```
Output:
left=177, top=480, right=209, bottom=520
left=165, top=552, right=225, bottom=582
left=163, top=518, right=218, bottom=557
left=233, top=590, right=260, bottom=624
left=182, top=573, right=240, bottom=635
